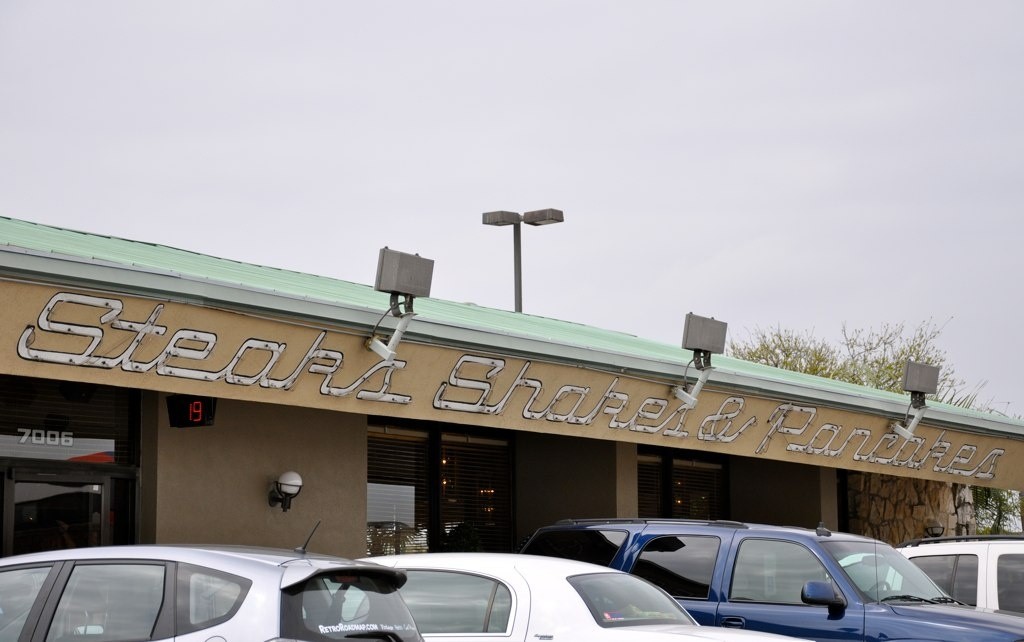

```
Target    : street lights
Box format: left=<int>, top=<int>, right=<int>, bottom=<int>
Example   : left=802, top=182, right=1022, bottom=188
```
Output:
left=481, top=209, right=564, bottom=314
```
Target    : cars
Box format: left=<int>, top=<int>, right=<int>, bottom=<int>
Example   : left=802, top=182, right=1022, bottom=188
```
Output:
left=0, top=543, right=427, bottom=641
left=320, top=552, right=821, bottom=642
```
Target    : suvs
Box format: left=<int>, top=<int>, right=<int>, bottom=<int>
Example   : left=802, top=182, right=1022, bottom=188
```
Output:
left=522, top=517, right=1024, bottom=642
left=835, top=533, right=1024, bottom=614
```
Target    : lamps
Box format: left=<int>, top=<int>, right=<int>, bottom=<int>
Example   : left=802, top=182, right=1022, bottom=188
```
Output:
left=671, top=312, right=728, bottom=408
left=366, top=246, right=434, bottom=362
left=925, top=519, right=944, bottom=537
left=269, top=471, right=303, bottom=512
left=892, top=360, right=940, bottom=441
